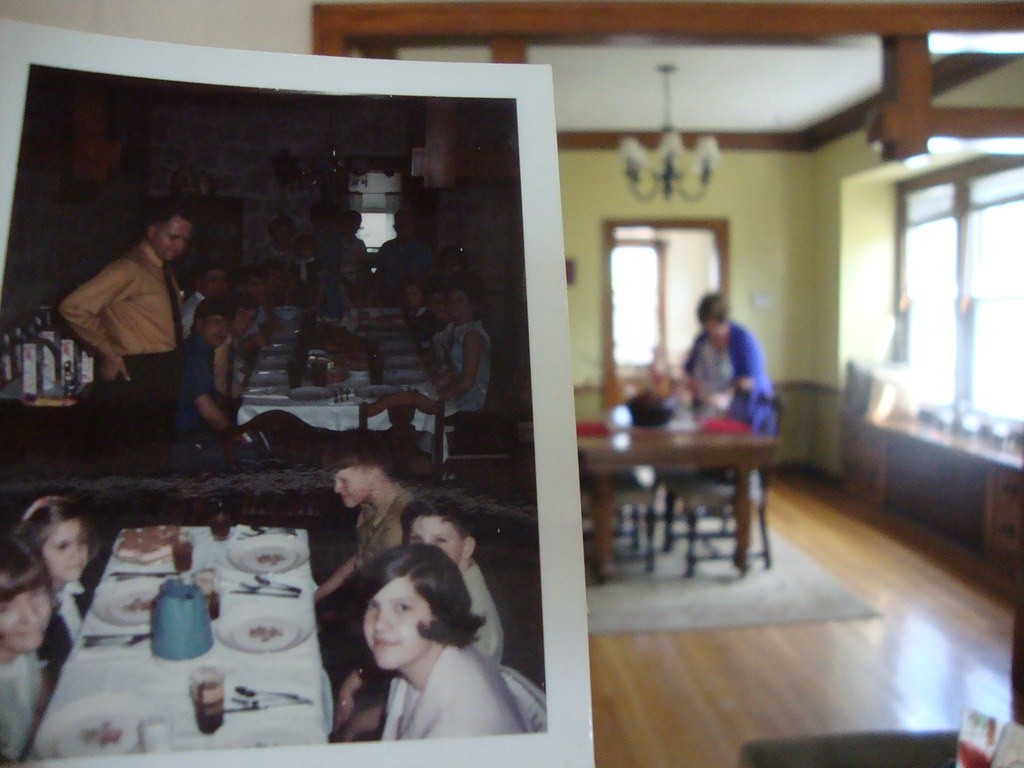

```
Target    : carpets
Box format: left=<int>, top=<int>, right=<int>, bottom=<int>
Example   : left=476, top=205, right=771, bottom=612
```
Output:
left=585, top=515, right=882, bottom=634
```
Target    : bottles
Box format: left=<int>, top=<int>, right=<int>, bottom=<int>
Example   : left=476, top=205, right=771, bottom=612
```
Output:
left=1, top=310, right=94, bottom=403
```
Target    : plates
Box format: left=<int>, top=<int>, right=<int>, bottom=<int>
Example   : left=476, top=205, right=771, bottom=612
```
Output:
left=210, top=711, right=328, bottom=748
left=245, top=304, right=428, bottom=405
left=218, top=605, right=311, bottom=652
left=92, top=579, right=158, bottom=625
left=112, top=525, right=192, bottom=561
left=229, top=536, right=310, bottom=572
left=35, top=694, right=142, bottom=759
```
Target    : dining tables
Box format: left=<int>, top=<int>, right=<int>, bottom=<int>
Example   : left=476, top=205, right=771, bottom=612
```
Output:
left=237, top=308, right=441, bottom=471
left=0, top=350, right=102, bottom=421
left=26, top=525, right=327, bottom=761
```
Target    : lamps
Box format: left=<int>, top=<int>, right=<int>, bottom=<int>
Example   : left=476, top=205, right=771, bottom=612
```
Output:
left=618, top=63, right=718, bottom=203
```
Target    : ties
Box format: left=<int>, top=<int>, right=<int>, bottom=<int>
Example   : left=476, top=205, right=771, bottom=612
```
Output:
left=164, top=262, right=185, bottom=352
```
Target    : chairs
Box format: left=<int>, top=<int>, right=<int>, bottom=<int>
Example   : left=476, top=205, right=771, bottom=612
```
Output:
left=662, top=391, right=787, bottom=577
left=576, top=466, right=657, bottom=571
left=650, top=385, right=754, bottom=552
left=0, top=297, right=520, bottom=472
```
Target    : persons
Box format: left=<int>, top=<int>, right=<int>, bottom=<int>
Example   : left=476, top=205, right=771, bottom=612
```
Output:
left=0, top=495, right=101, bottom=768
left=59, top=205, right=197, bottom=477
left=684, top=293, right=777, bottom=437
left=311, top=443, right=523, bottom=743
left=170, top=200, right=493, bottom=473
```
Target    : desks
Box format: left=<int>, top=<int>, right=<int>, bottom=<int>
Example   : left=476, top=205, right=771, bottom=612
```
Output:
left=578, top=403, right=786, bottom=582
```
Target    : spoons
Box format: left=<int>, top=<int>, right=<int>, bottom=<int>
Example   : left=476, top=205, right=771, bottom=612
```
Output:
left=255, top=575, right=301, bottom=592
left=235, top=686, right=299, bottom=700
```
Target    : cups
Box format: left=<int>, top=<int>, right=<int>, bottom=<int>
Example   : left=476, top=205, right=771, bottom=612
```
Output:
left=171, top=527, right=194, bottom=572
left=211, top=498, right=231, bottom=540
left=189, top=667, right=224, bottom=735
left=138, top=716, right=174, bottom=753
left=190, top=568, right=222, bottom=619
